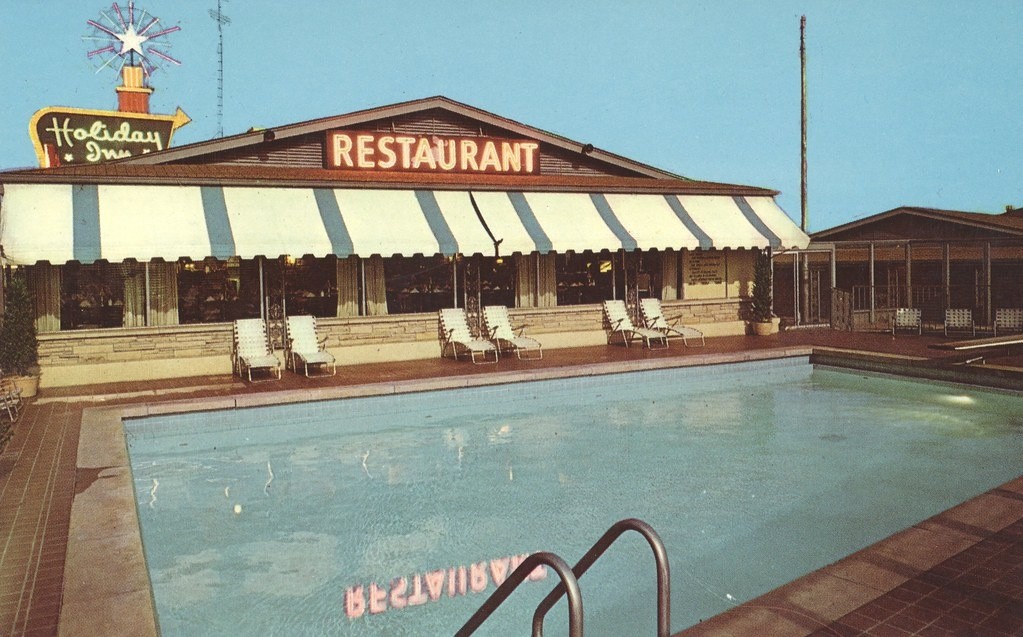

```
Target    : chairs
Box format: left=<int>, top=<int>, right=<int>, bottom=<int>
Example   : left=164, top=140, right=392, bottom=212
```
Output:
left=603, top=300, right=670, bottom=351
left=993, top=308, right=1023, bottom=338
left=437, top=308, right=500, bottom=364
left=233, top=319, right=282, bottom=383
left=639, top=298, right=706, bottom=348
left=892, top=308, right=921, bottom=336
left=286, top=315, right=336, bottom=377
left=481, top=306, right=543, bottom=361
left=0, top=380, right=24, bottom=423
left=944, top=309, right=975, bottom=338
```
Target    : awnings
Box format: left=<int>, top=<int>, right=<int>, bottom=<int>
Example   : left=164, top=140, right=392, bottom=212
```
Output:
left=0, top=182, right=811, bottom=271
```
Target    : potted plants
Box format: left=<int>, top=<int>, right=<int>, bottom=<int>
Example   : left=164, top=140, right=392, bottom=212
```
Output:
left=747, top=248, right=773, bottom=337
left=0, top=268, right=41, bottom=398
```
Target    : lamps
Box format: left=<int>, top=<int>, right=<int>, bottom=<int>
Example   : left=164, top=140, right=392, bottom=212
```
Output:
left=584, top=142, right=595, bottom=153
left=263, top=128, right=275, bottom=143
